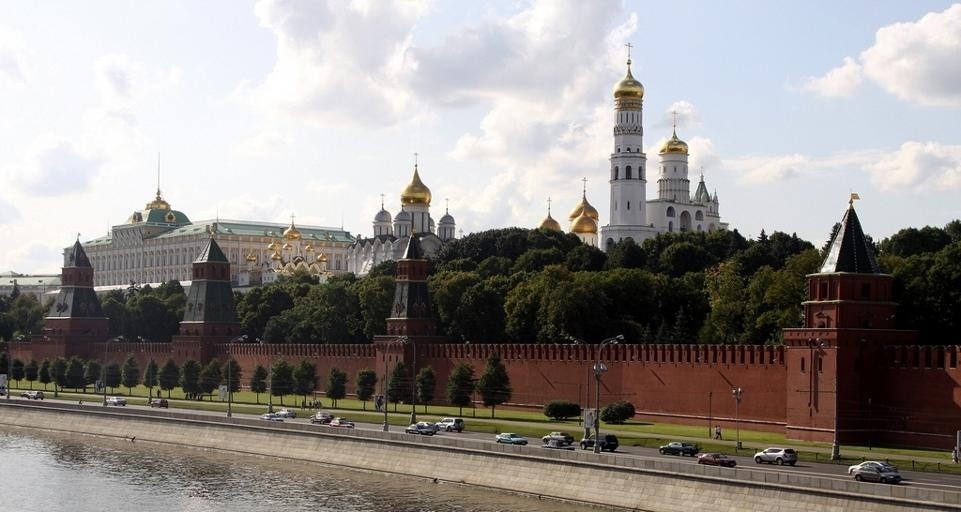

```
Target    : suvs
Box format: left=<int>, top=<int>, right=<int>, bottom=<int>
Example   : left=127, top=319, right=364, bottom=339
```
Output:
left=580, top=433, right=618, bottom=451
left=405, top=418, right=464, bottom=435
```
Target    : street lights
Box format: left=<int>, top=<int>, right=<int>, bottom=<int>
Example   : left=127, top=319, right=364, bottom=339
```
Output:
left=227, top=335, right=249, bottom=416
left=585, top=335, right=624, bottom=454
left=732, top=387, right=742, bottom=451
left=255, top=338, right=273, bottom=413
left=382, top=338, right=417, bottom=431
left=44, top=336, right=57, bottom=397
left=7, top=335, right=25, bottom=399
left=138, top=336, right=152, bottom=406
left=103, top=336, right=124, bottom=406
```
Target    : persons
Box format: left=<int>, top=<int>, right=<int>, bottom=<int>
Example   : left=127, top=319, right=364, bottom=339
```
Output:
left=299, top=398, right=321, bottom=412
left=712, top=424, right=719, bottom=440
left=715, top=425, right=722, bottom=441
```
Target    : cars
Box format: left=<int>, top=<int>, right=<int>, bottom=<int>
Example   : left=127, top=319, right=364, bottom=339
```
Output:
left=659, top=442, right=699, bottom=456
left=151, top=399, right=168, bottom=408
left=848, top=460, right=902, bottom=484
left=754, top=446, right=798, bottom=465
left=496, top=433, right=528, bottom=445
left=21, top=391, right=44, bottom=400
left=259, top=409, right=297, bottom=421
left=106, top=397, right=128, bottom=406
left=698, top=453, right=736, bottom=467
left=310, top=411, right=354, bottom=428
left=542, top=432, right=574, bottom=445
